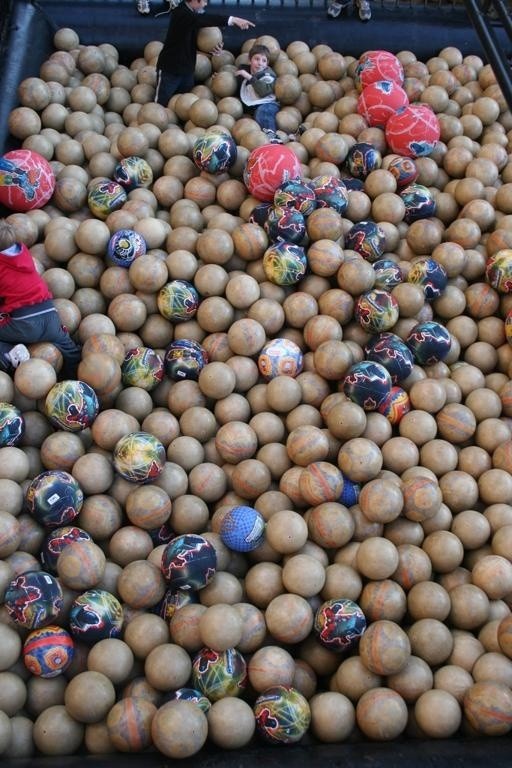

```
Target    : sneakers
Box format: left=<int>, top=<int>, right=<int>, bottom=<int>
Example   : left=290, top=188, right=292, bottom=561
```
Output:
left=328, top=2, right=348, bottom=17
left=355, top=1, right=371, bottom=20
left=267, top=130, right=283, bottom=144
left=138, top=0, right=150, bottom=13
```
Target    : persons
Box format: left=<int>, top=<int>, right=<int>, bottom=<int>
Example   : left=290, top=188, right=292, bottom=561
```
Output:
left=328, top=0, right=372, bottom=20
left=1, top=220, right=80, bottom=382
left=235, top=45, right=280, bottom=133
left=155, top=0, right=255, bottom=112
left=136, top=0, right=181, bottom=17
